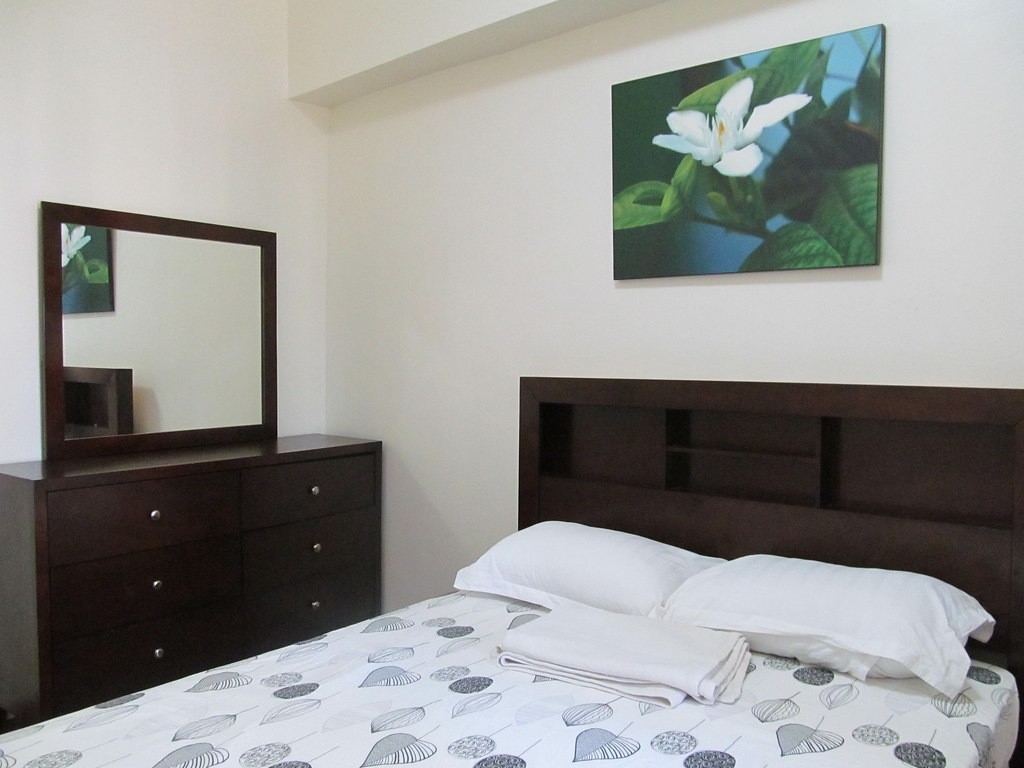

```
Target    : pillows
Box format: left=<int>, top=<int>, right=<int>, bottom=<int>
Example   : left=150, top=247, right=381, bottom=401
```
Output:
left=452, top=520, right=730, bottom=617
left=648, top=553, right=997, bottom=700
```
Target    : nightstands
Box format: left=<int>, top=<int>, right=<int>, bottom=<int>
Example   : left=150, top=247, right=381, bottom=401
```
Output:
left=0, top=432, right=382, bottom=735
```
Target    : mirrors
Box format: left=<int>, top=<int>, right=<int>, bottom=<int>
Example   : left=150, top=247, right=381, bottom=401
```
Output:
left=38, top=197, right=279, bottom=460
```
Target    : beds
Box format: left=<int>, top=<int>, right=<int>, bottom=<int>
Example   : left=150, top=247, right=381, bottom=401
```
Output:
left=0, top=374, right=1024, bottom=768
